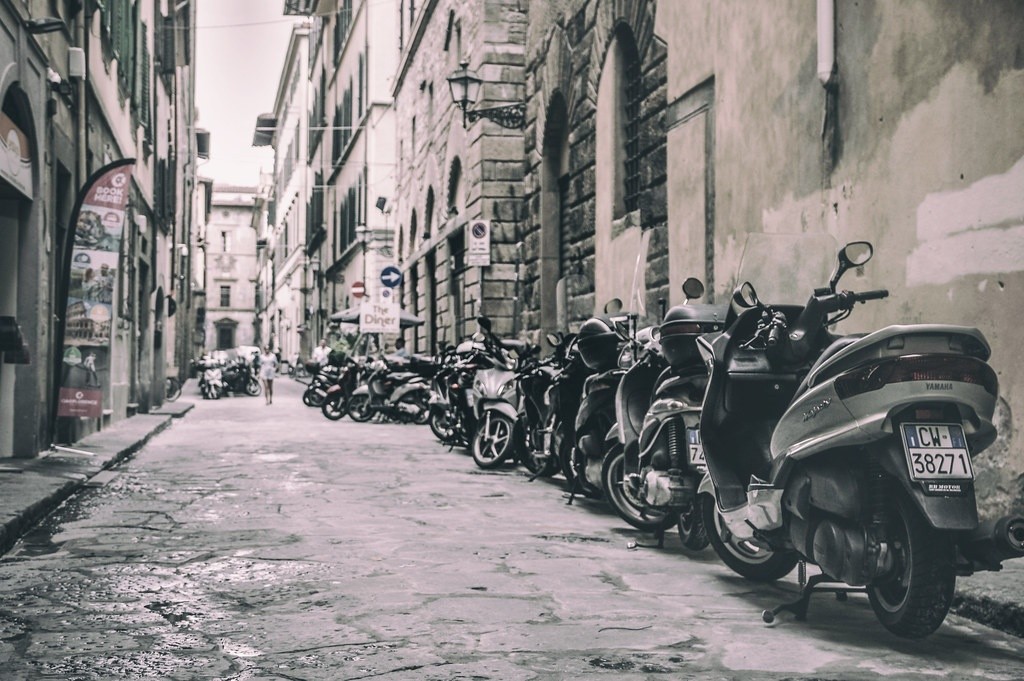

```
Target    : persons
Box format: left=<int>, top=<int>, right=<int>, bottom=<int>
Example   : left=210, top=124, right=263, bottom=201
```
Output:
left=393, top=337, right=406, bottom=358
left=311, top=339, right=333, bottom=384
left=257, top=345, right=279, bottom=405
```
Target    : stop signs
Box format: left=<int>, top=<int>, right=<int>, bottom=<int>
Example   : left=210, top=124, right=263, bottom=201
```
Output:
left=352, top=282, right=365, bottom=297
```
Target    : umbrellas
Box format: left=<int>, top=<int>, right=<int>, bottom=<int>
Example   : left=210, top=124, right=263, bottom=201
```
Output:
left=329, top=305, right=425, bottom=365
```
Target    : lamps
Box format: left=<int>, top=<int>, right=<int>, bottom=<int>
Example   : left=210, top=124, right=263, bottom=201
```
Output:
left=445, top=56, right=525, bottom=132
left=26, top=16, right=65, bottom=34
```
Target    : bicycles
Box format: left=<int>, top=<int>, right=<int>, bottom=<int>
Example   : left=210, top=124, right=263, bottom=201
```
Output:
left=165, top=378, right=182, bottom=401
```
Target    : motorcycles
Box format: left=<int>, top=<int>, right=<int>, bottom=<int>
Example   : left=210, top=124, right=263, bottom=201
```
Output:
left=190, top=353, right=262, bottom=400
left=302, top=277, right=725, bottom=551
left=698, top=240, right=1024, bottom=640
